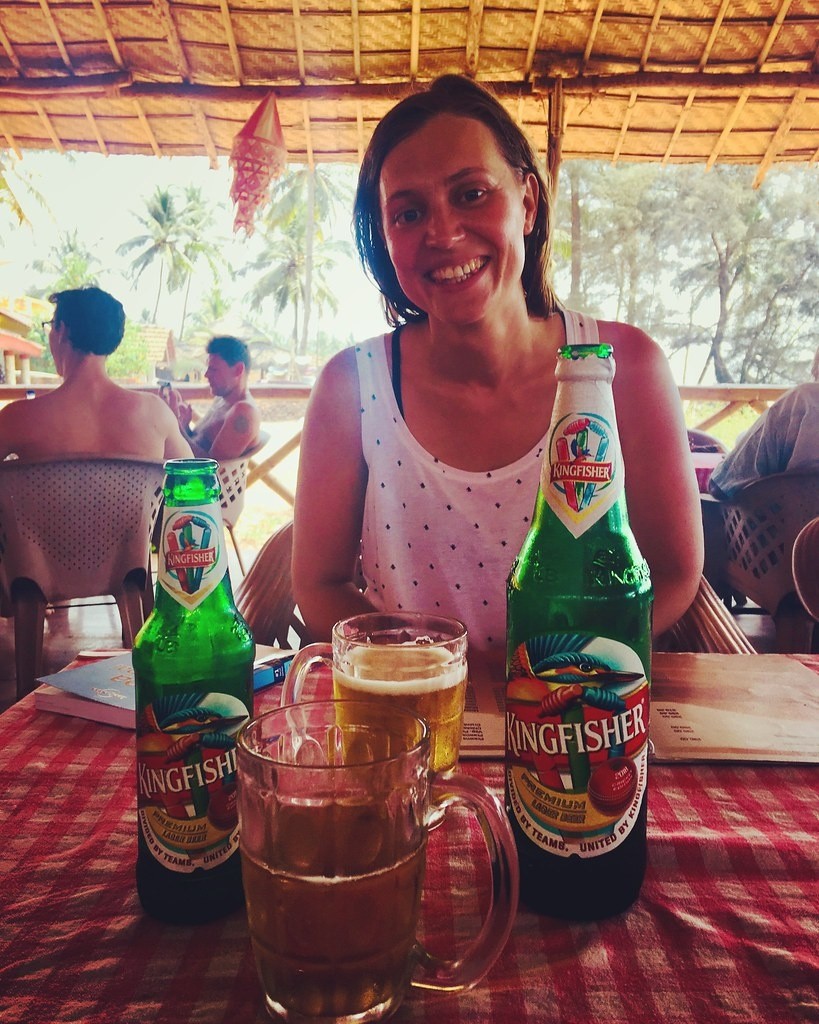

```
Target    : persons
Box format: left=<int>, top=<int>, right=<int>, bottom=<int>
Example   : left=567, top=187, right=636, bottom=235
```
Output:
left=707, top=346, right=819, bottom=619
left=151, top=335, right=261, bottom=553
left=1, top=283, right=200, bottom=612
left=288, top=71, right=706, bottom=653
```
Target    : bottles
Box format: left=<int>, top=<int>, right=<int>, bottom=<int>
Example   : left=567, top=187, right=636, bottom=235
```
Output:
left=131, top=458, right=255, bottom=924
left=505, top=345, right=654, bottom=920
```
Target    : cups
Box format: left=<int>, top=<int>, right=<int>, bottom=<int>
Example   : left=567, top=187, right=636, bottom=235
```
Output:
left=282, top=611, right=468, bottom=780
left=237, top=699, right=519, bottom=1024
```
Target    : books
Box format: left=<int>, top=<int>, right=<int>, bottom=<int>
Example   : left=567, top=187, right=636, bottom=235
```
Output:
left=34, top=643, right=294, bottom=729
left=459, top=652, right=819, bottom=767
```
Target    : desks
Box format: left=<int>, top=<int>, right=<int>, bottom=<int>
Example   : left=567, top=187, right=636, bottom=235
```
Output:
left=692, top=452, right=731, bottom=493
left=0, top=606, right=819, bottom=1024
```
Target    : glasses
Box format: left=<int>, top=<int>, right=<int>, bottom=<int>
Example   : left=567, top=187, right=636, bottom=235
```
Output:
left=42, top=320, right=55, bottom=337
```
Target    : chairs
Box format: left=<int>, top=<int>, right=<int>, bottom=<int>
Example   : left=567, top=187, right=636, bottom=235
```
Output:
left=202, top=430, right=271, bottom=577
left=233, top=518, right=311, bottom=650
left=0, top=452, right=167, bottom=702
left=657, top=463, right=818, bottom=655
